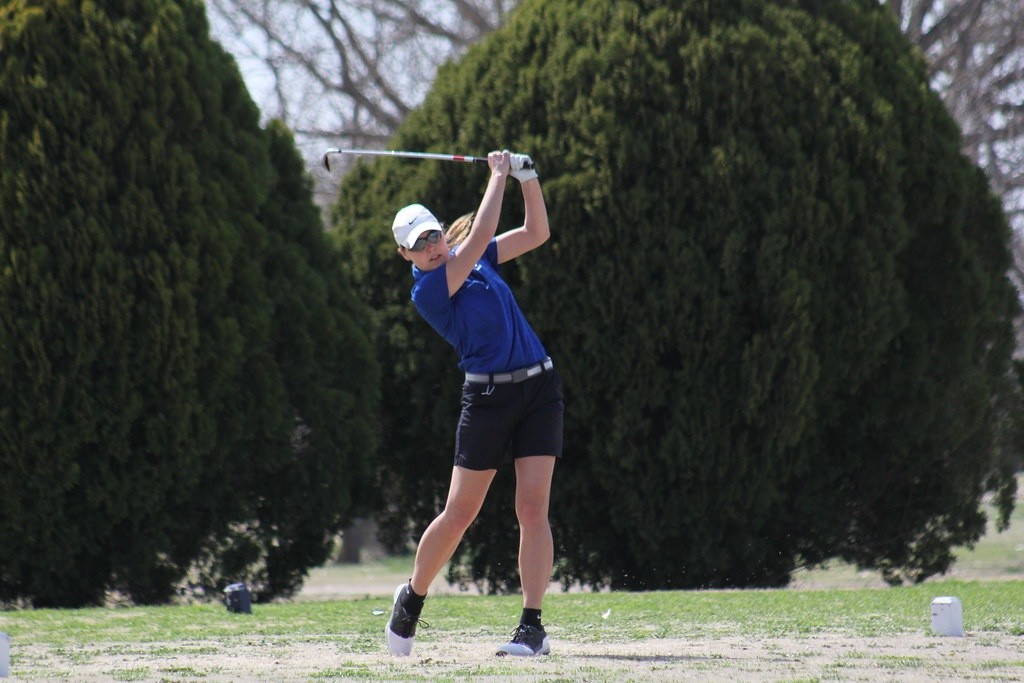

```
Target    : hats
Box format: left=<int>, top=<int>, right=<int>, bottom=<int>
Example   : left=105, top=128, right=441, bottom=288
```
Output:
left=392, top=203, right=442, bottom=249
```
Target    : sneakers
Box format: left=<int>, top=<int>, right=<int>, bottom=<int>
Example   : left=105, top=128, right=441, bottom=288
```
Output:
left=386, top=583, right=425, bottom=657
left=496, top=625, right=551, bottom=657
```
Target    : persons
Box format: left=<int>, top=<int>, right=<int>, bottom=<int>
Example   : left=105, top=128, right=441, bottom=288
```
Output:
left=384, top=150, right=563, bottom=656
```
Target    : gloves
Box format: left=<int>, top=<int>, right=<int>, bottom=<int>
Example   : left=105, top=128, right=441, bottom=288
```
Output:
left=508, top=153, right=538, bottom=182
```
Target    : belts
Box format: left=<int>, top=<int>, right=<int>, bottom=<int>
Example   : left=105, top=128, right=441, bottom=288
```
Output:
left=464, top=357, right=554, bottom=383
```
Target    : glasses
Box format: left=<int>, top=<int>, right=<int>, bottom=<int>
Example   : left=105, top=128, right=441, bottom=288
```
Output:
left=402, top=229, right=441, bottom=252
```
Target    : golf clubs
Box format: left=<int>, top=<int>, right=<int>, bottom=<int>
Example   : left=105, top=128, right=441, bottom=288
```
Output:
left=321, top=147, right=536, bottom=173
